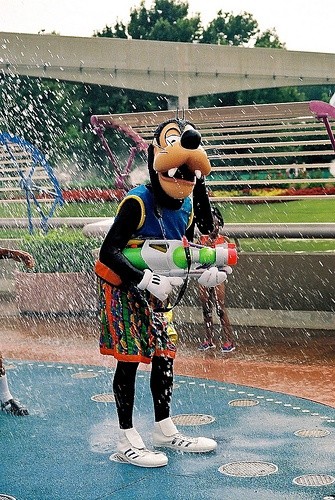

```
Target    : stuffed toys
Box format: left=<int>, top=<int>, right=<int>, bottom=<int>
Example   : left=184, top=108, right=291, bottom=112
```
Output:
left=94, top=106, right=232, bottom=468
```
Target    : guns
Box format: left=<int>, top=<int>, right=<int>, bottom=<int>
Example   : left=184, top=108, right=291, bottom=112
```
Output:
left=121, top=237, right=246, bottom=304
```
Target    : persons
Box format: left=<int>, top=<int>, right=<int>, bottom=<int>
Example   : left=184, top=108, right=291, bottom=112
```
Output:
left=194, top=214, right=237, bottom=353
left=285, top=160, right=311, bottom=179
left=0, top=246, right=36, bottom=416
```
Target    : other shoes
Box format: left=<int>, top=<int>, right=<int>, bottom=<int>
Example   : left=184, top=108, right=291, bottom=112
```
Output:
left=1, top=398, right=29, bottom=416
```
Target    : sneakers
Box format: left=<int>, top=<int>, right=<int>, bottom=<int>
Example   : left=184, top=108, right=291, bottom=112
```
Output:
left=151, top=418, right=217, bottom=452
left=221, top=342, right=236, bottom=352
left=197, top=341, right=216, bottom=350
left=117, top=424, right=168, bottom=467
